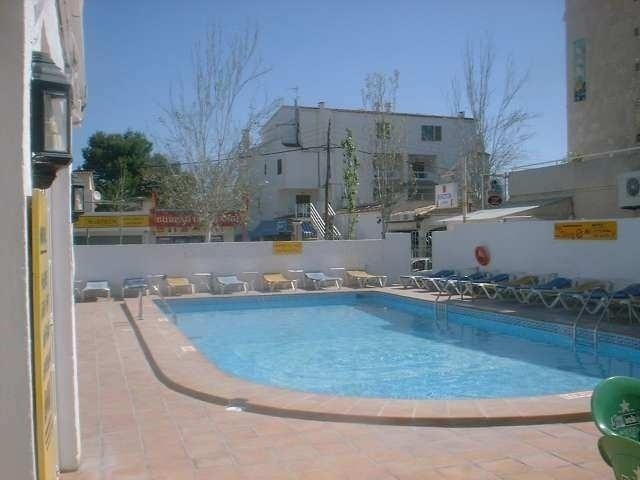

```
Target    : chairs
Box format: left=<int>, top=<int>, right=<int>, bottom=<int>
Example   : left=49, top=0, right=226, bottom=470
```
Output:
left=216, top=275, right=247, bottom=294
left=81, top=278, right=112, bottom=302
left=400, top=267, right=640, bottom=324
left=346, top=270, right=385, bottom=289
left=121, top=278, right=147, bottom=297
left=262, top=272, right=296, bottom=293
left=167, top=278, right=195, bottom=296
left=590, top=374, right=639, bottom=445
left=305, top=272, right=342, bottom=290
left=598, top=433, right=639, bottom=480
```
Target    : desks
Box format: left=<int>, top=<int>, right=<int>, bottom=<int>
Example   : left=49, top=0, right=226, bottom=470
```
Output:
left=329, top=268, right=345, bottom=287
left=192, top=272, right=212, bottom=293
left=145, top=273, right=166, bottom=297
left=242, top=271, right=258, bottom=292
left=287, top=270, right=303, bottom=288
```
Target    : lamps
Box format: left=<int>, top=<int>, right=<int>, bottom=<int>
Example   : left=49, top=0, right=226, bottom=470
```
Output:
left=32, top=51, right=73, bottom=192
left=71, top=172, right=86, bottom=223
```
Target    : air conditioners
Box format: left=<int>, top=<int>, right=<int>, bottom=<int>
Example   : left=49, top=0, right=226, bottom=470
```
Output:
left=616, top=169, right=640, bottom=211
